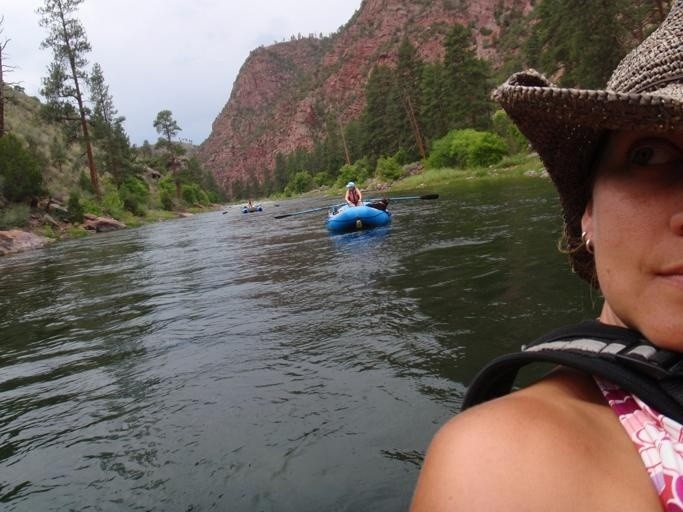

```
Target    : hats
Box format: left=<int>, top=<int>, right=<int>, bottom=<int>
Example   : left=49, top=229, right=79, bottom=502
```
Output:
left=346, top=182, right=355, bottom=188
left=489, top=2, right=683, bottom=284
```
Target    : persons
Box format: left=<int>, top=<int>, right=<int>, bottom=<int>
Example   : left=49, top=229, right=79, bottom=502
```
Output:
left=405, top=0, right=682, bottom=510
left=344, top=181, right=362, bottom=208
left=247, top=199, right=252, bottom=208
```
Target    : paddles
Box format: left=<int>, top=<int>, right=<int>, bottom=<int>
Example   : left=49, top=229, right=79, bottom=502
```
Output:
left=222, top=204, right=280, bottom=215
left=275, top=202, right=347, bottom=219
left=362, top=194, right=439, bottom=202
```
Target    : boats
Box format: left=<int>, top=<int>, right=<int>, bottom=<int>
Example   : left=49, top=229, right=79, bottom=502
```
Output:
left=322, top=199, right=395, bottom=234
left=242, top=204, right=265, bottom=215
